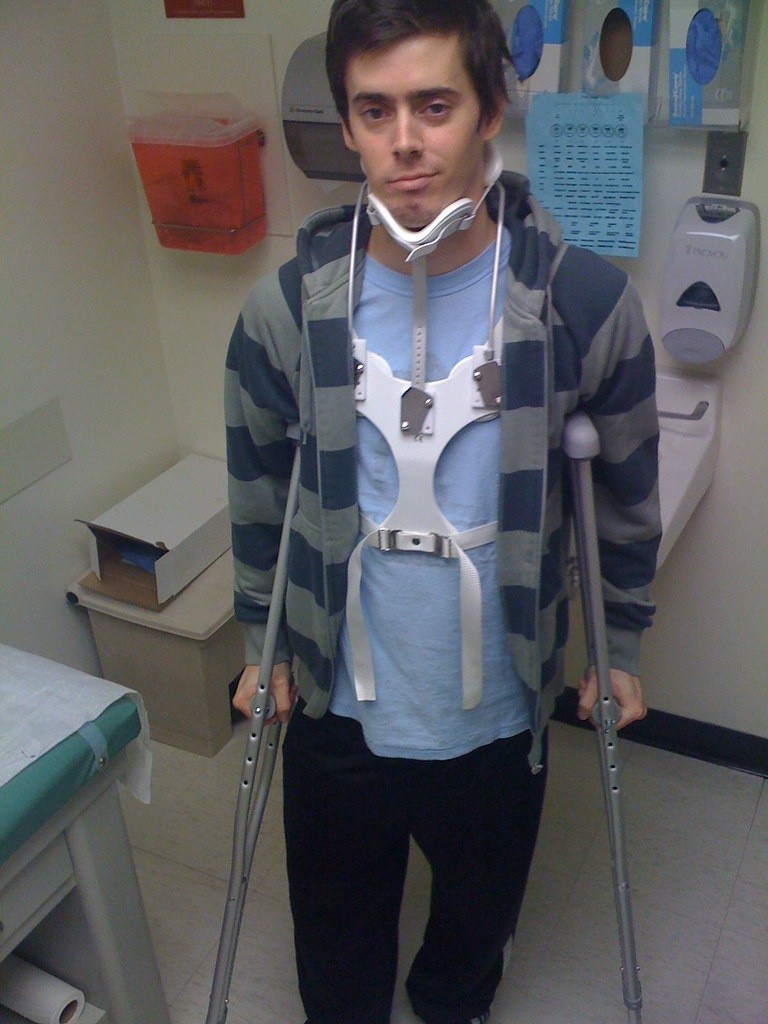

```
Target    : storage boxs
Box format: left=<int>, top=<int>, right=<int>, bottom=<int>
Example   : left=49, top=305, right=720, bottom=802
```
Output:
left=129, top=91, right=266, bottom=255
left=73, top=452, right=233, bottom=611
left=87, top=547, right=247, bottom=758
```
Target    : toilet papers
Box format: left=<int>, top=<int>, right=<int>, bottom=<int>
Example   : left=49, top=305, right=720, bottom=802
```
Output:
left=0, top=955, right=85, bottom=1023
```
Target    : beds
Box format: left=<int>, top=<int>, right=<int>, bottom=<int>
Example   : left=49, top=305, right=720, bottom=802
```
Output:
left=0, top=641, right=172, bottom=1024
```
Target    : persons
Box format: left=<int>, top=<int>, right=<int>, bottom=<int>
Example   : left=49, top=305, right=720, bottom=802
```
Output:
left=222, top=0, right=662, bottom=1024
